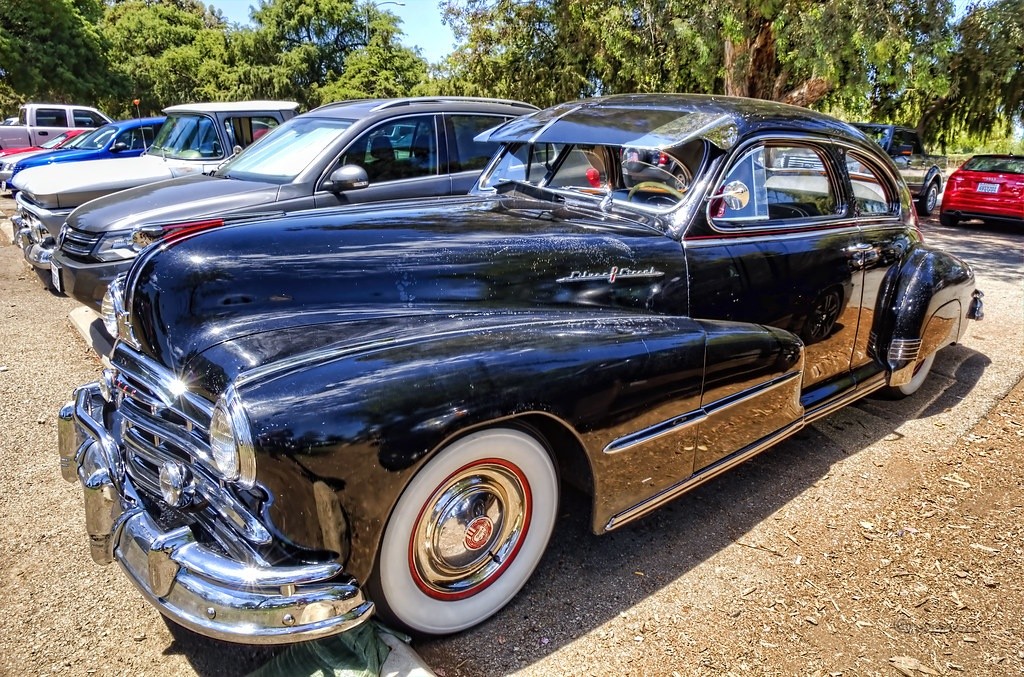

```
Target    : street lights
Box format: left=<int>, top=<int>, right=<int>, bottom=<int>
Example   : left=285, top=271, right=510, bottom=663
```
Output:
left=366, top=1, right=406, bottom=45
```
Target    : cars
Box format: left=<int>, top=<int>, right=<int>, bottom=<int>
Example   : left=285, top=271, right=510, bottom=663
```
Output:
left=0, top=129, right=99, bottom=162
left=1, top=116, right=221, bottom=199
left=224, top=117, right=418, bottom=160
left=940, top=154, right=1024, bottom=228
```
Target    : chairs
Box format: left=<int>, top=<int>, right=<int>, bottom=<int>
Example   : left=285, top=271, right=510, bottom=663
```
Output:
left=390, top=134, right=430, bottom=179
left=366, top=137, right=396, bottom=180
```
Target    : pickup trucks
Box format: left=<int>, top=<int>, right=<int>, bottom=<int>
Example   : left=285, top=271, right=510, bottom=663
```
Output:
left=0, top=102, right=136, bottom=151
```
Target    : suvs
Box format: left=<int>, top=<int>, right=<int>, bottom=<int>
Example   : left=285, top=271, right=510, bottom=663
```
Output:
left=55, top=92, right=984, bottom=646
left=769, top=122, right=943, bottom=217
left=10, top=100, right=304, bottom=271
left=50, top=95, right=601, bottom=324
